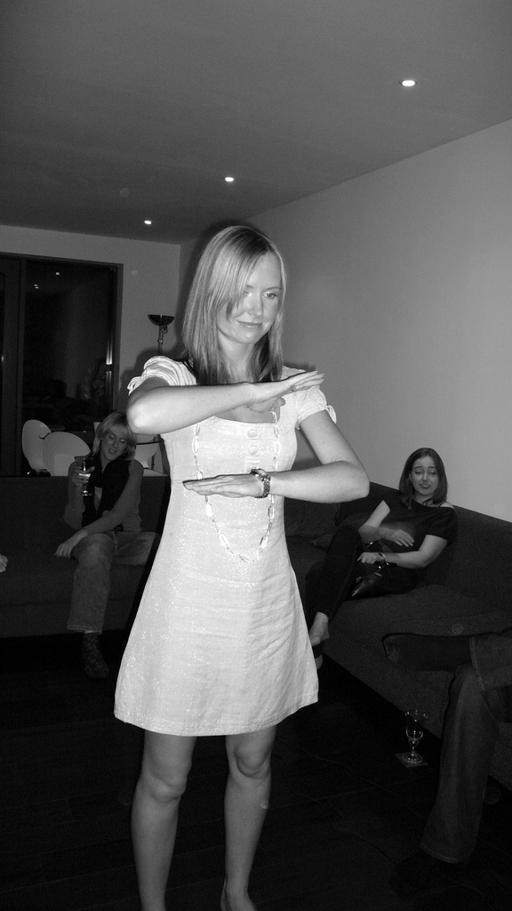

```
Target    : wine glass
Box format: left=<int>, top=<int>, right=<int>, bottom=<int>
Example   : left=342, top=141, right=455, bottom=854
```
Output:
left=74, top=456, right=95, bottom=497
left=399, top=708, right=428, bottom=767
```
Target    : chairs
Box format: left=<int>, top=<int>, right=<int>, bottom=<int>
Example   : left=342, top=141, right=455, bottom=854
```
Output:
left=20, top=418, right=52, bottom=476
left=40, top=430, right=93, bottom=479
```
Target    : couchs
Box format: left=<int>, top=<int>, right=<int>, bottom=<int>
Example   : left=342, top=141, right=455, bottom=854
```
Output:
left=284, top=481, right=512, bottom=791
left=0, top=476, right=171, bottom=643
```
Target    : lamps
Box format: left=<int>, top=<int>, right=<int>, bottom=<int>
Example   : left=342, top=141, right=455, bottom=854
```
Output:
left=148, top=313, right=176, bottom=354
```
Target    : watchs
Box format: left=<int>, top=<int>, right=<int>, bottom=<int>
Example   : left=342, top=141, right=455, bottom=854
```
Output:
left=249, top=468, right=271, bottom=499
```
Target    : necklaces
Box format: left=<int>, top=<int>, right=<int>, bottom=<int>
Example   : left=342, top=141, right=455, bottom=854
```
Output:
left=191, top=410, right=281, bottom=561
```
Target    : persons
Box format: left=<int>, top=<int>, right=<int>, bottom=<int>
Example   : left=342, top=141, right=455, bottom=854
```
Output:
left=51, top=410, right=157, bottom=680
left=302, top=447, right=458, bottom=669
left=114, top=226, right=370, bottom=910
left=382, top=626, right=512, bottom=899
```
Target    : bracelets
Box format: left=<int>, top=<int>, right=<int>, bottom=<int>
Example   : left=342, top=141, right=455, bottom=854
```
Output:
left=377, top=552, right=387, bottom=562
